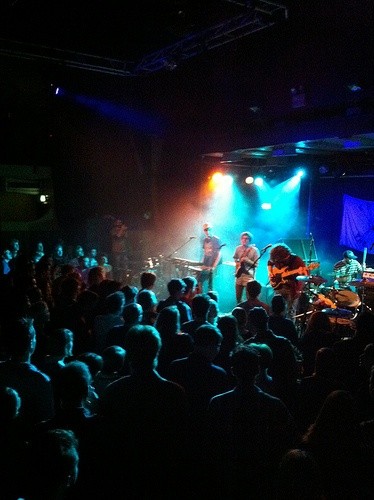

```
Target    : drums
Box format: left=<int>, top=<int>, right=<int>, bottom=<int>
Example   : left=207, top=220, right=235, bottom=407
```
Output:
left=335, top=290, right=360, bottom=308
left=329, top=317, right=354, bottom=335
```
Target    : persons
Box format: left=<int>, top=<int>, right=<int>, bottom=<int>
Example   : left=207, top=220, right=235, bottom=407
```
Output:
left=111, top=218, right=128, bottom=278
left=0, top=224, right=374, bottom=500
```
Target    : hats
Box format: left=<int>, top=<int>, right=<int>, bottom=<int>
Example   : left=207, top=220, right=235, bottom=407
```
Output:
left=343, top=250, right=357, bottom=259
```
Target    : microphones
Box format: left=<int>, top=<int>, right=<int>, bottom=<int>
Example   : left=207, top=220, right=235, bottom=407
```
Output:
left=190, top=236, right=197, bottom=239
left=263, top=244, right=272, bottom=251
left=219, top=243, right=226, bottom=249
left=310, top=232, right=314, bottom=242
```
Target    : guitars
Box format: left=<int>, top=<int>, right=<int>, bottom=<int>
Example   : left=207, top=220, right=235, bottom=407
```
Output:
left=235, top=244, right=256, bottom=279
left=268, top=263, right=320, bottom=289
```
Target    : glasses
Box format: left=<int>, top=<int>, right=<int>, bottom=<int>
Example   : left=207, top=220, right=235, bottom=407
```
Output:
left=203, top=227, right=209, bottom=232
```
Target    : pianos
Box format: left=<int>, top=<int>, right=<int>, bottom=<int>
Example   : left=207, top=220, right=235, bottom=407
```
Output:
left=169, top=258, right=212, bottom=273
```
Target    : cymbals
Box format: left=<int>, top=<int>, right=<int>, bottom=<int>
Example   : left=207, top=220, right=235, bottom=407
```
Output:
left=295, top=274, right=327, bottom=284
left=349, top=280, right=374, bottom=288
